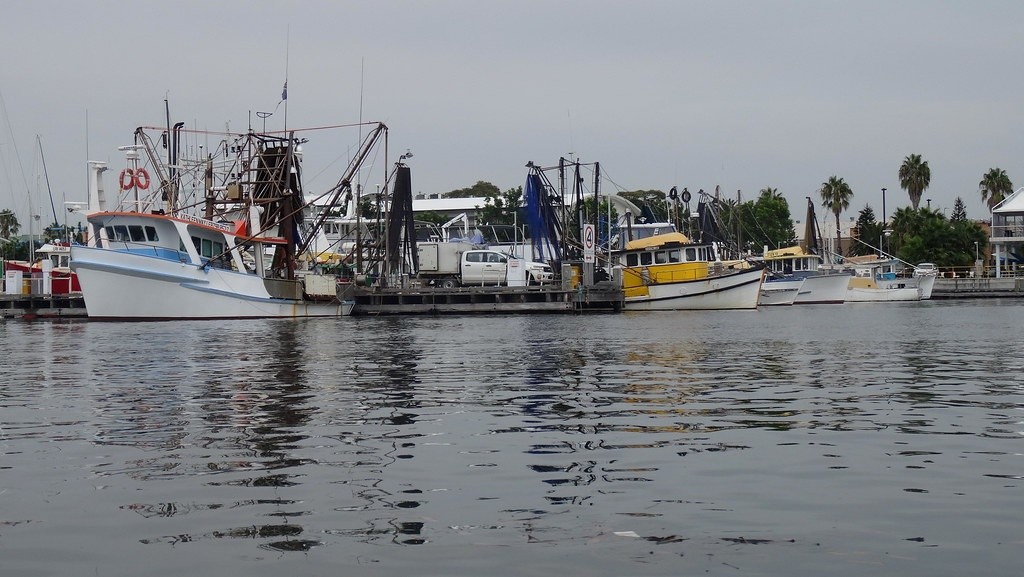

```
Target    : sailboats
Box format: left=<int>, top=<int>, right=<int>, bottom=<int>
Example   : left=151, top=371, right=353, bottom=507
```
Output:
left=0, top=41, right=1021, bottom=309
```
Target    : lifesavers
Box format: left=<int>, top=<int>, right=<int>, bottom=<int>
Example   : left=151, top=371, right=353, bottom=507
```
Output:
left=120, top=168, right=134, bottom=190
left=135, top=168, right=150, bottom=189
